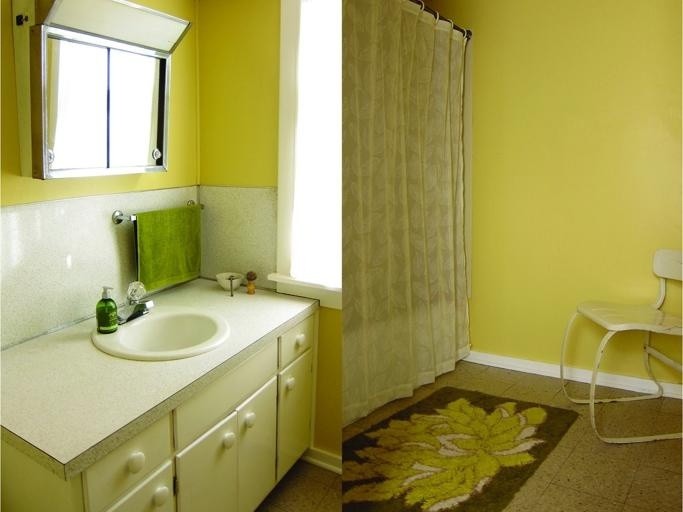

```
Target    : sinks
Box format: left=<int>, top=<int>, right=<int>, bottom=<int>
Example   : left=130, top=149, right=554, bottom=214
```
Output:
left=92, top=308, right=229, bottom=361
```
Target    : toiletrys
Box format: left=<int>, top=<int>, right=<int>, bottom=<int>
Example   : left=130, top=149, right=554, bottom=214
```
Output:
left=246, top=271, right=257, bottom=296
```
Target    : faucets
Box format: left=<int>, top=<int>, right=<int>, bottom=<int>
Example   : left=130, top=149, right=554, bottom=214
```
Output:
left=117, top=281, right=154, bottom=323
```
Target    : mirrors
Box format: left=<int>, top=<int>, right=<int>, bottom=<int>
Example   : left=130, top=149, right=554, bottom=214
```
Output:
left=29, top=23, right=172, bottom=180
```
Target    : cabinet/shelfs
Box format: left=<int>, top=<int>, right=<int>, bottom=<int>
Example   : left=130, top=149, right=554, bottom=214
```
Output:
left=2, top=313, right=321, bottom=512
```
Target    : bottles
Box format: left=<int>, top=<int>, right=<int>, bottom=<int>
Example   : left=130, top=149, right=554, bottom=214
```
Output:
left=247, top=280, right=256, bottom=294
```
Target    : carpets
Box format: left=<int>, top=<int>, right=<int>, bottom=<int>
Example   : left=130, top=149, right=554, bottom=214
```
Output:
left=340, top=386, right=578, bottom=510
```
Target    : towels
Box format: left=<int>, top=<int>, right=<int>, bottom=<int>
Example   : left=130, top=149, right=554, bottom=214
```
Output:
left=133, top=204, right=206, bottom=294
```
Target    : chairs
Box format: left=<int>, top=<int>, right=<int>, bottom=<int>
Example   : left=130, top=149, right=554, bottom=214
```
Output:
left=558, top=248, right=683, bottom=446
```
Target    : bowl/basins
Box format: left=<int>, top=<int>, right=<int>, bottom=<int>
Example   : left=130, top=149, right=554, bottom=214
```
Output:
left=216, top=272, right=245, bottom=291
